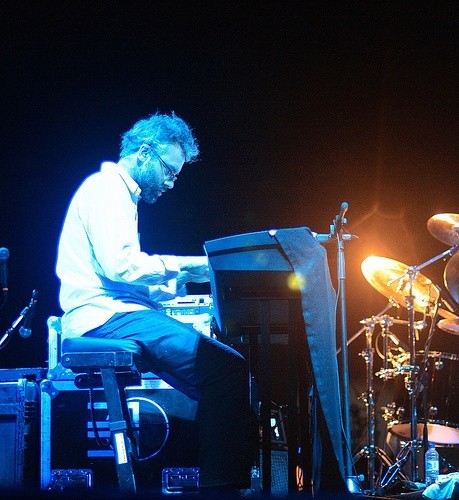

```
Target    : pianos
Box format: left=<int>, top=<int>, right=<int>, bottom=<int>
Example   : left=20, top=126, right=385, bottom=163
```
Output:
left=203, top=224, right=353, bottom=500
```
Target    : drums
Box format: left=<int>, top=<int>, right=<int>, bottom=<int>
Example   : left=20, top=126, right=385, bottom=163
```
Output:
left=384, top=423, right=459, bottom=483
left=386, top=350, right=459, bottom=428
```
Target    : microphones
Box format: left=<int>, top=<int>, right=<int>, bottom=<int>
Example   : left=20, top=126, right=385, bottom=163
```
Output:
left=19, top=290, right=38, bottom=338
left=0, top=247, right=9, bottom=302
left=433, top=280, right=459, bottom=317
left=312, top=231, right=360, bottom=243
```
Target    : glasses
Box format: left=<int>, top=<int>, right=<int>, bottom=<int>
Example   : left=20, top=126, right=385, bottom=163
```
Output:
left=148, top=144, right=177, bottom=181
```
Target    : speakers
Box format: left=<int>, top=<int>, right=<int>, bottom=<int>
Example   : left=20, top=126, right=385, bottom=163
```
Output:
left=251, top=443, right=290, bottom=500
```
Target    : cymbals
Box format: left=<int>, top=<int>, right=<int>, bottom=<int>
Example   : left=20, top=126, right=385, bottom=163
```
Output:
left=444, top=249, right=459, bottom=308
left=361, top=256, right=443, bottom=316
left=426, top=212, right=459, bottom=249
left=437, top=319, right=459, bottom=337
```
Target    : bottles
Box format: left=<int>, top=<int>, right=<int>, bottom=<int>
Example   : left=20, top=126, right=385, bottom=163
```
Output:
left=425, top=444, right=439, bottom=489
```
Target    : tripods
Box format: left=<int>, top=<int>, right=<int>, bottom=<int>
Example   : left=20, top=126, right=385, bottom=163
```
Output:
left=346, top=243, right=459, bottom=496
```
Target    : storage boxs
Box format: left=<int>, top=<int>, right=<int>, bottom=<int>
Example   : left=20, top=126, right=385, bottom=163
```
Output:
left=47, top=312, right=213, bottom=383
left=39, top=380, right=198, bottom=495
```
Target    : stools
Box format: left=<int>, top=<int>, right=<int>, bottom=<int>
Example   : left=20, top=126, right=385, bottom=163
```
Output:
left=61, top=334, right=151, bottom=497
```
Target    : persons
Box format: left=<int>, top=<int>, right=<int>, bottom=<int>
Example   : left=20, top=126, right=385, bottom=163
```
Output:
left=56, top=111, right=251, bottom=494
